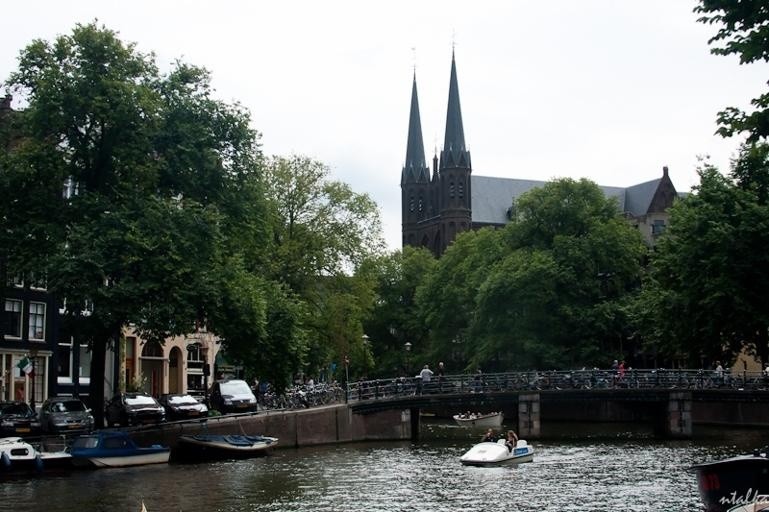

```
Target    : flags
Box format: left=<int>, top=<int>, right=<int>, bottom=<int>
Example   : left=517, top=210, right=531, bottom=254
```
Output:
left=17, top=353, right=36, bottom=380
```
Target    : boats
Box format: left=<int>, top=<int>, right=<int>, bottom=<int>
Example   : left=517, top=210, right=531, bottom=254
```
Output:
left=63, top=432, right=171, bottom=466
left=179, top=431, right=280, bottom=459
left=453, top=410, right=506, bottom=430
left=0, top=437, right=72, bottom=476
left=682, top=446, right=769, bottom=512
left=460, top=438, right=535, bottom=469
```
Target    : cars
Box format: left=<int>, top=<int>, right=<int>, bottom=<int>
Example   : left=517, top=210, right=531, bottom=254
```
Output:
left=105, top=392, right=168, bottom=427
left=1, top=401, right=42, bottom=439
left=158, top=394, right=209, bottom=424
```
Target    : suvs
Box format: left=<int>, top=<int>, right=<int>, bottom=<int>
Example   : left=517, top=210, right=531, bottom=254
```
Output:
left=209, top=378, right=258, bottom=416
left=39, top=395, right=95, bottom=433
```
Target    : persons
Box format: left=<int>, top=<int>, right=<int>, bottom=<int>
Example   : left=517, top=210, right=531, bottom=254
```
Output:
left=609, top=358, right=619, bottom=376
left=16, top=385, right=26, bottom=402
left=418, top=363, right=434, bottom=384
left=481, top=427, right=498, bottom=443
left=304, top=374, right=315, bottom=388
left=458, top=409, right=499, bottom=421
left=474, top=368, right=488, bottom=387
left=703, top=359, right=723, bottom=390
left=619, top=359, right=627, bottom=376
left=506, top=429, right=519, bottom=454
left=761, top=361, right=769, bottom=378
left=435, top=361, right=449, bottom=378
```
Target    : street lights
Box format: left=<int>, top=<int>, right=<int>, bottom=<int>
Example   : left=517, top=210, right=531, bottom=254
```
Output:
left=360, top=333, right=370, bottom=379
left=404, top=340, right=412, bottom=377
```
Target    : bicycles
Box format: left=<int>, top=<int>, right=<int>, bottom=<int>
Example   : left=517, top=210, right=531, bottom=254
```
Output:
left=261, top=380, right=342, bottom=412
